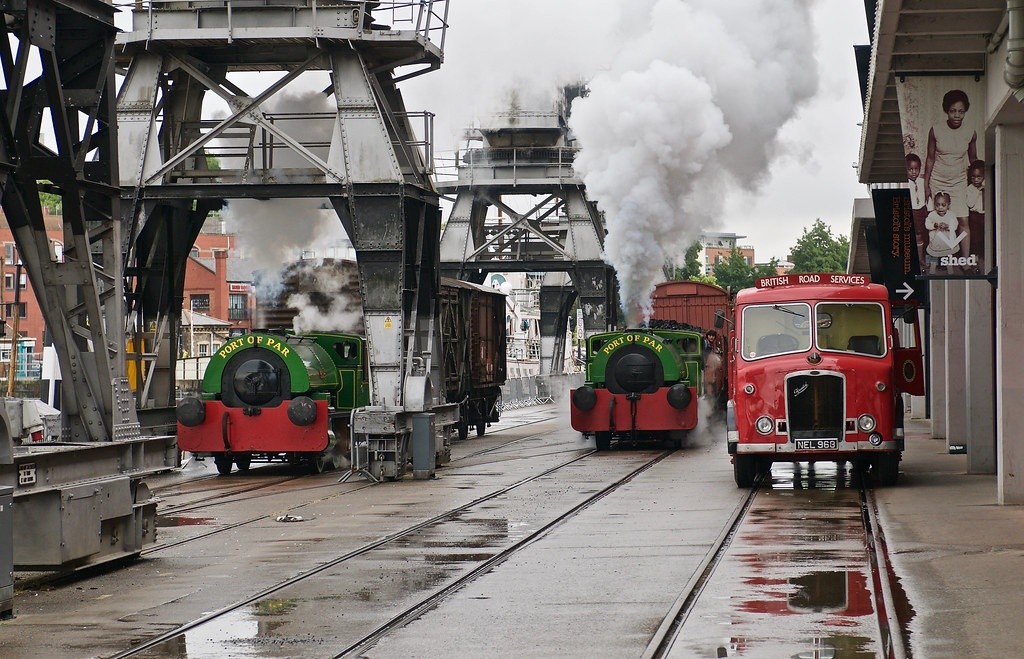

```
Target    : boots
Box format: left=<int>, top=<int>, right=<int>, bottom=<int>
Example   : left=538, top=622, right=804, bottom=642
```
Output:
left=947, top=263, right=954, bottom=274
left=929, top=261, right=936, bottom=275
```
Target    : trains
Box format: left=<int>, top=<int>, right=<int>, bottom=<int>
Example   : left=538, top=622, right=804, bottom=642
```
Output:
left=569, top=278, right=738, bottom=451
left=725, top=273, right=923, bottom=489
left=178, top=259, right=521, bottom=472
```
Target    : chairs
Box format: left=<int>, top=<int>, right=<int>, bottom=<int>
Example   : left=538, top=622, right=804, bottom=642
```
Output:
left=757, top=333, right=800, bottom=367
left=848, top=335, right=881, bottom=364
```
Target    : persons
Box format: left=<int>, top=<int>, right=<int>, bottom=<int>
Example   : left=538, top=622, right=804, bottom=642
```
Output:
left=906, top=89, right=985, bottom=278
left=703, top=330, right=717, bottom=364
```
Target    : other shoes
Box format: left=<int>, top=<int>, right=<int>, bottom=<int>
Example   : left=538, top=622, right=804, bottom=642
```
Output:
left=960, top=264, right=975, bottom=276
left=925, top=263, right=931, bottom=272
left=922, top=269, right=927, bottom=274
